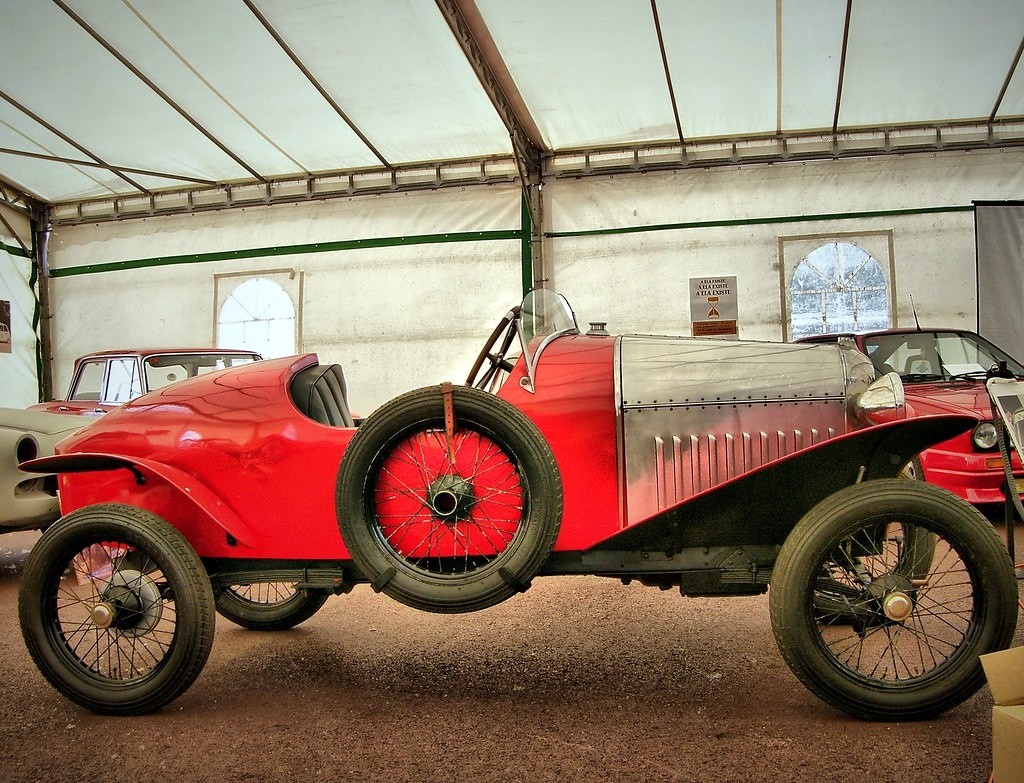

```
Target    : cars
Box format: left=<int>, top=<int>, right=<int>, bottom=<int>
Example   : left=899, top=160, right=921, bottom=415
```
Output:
left=25, top=348, right=263, bottom=417
left=795, top=327, right=1024, bottom=504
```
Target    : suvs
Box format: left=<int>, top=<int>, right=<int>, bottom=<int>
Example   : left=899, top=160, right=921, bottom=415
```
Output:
left=17, top=290, right=1018, bottom=722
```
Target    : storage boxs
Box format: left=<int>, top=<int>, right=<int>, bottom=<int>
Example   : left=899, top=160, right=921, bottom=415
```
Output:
left=979, top=646, right=1024, bottom=782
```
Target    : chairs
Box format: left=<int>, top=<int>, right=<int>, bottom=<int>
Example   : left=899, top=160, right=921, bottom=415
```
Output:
left=883, top=364, right=896, bottom=373
left=291, top=364, right=357, bottom=430
left=904, top=355, right=934, bottom=376
